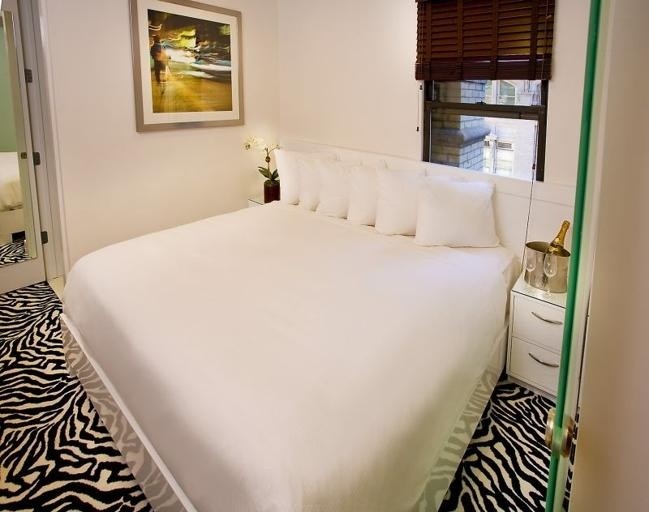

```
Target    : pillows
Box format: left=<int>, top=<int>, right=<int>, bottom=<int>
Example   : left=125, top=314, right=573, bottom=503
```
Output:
left=274, top=150, right=501, bottom=248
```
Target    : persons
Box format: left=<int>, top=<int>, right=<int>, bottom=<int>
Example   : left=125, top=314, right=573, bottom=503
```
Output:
left=150, top=35, right=163, bottom=85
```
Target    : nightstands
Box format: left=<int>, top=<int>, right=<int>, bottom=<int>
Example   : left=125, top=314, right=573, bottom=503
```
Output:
left=506, top=268, right=567, bottom=407
left=248, top=196, right=264, bottom=208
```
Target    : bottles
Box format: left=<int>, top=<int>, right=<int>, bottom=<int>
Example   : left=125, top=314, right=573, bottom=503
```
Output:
left=543, top=220, right=570, bottom=257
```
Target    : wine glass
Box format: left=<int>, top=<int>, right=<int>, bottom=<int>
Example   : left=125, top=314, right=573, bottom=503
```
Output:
left=543, top=253, right=557, bottom=299
left=524, top=248, right=537, bottom=293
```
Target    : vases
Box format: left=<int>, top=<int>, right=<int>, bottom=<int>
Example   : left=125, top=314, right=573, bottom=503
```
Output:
left=264, top=180, right=280, bottom=203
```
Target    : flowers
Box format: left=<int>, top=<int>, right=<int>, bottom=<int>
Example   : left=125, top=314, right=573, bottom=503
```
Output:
left=244, top=135, right=282, bottom=180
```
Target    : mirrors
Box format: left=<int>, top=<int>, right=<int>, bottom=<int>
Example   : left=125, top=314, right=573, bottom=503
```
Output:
left=0, top=9, right=38, bottom=266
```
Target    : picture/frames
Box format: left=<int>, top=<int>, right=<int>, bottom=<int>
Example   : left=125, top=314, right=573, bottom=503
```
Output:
left=128, top=0, right=244, bottom=132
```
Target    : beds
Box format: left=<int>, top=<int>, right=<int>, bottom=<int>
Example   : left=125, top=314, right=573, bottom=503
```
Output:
left=0, top=180, right=24, bottom=245
left=60, top=201, right=520, bottom=512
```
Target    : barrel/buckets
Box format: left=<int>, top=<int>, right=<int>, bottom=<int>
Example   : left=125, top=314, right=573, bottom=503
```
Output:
left=526, top=241, right=568, bottom=293
